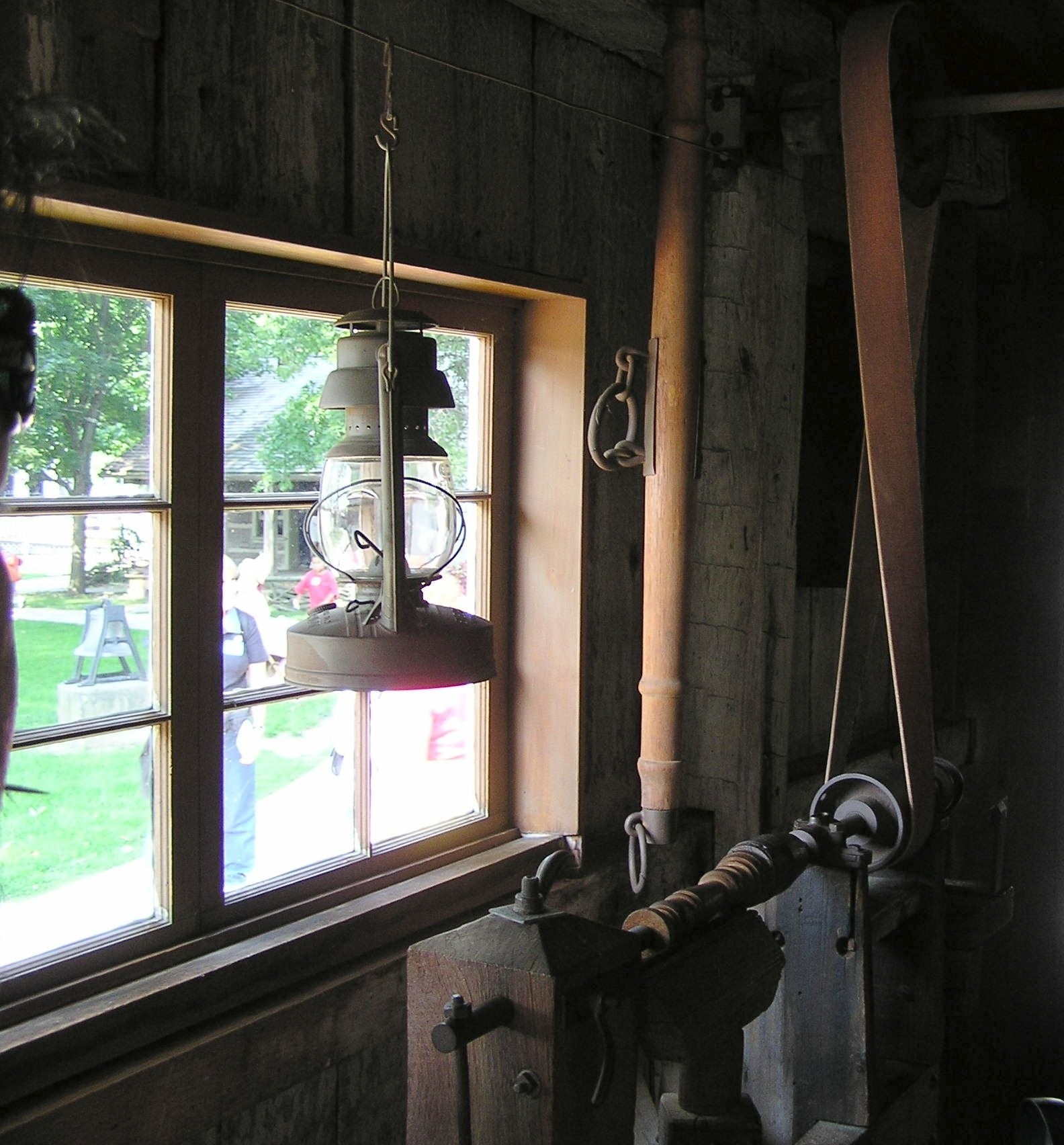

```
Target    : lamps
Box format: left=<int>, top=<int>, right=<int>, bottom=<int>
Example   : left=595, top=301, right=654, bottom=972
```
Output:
left=282, top=42, right=505, bottom=690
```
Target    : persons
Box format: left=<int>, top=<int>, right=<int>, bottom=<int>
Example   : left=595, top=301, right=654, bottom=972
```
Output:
left=222, top=554, right=269, bottom=887
left=331, top=690, right=380, bottom=776
left=293, top=555, right=340, bottom=609
left=0, top=545, right=24, bottom=590
